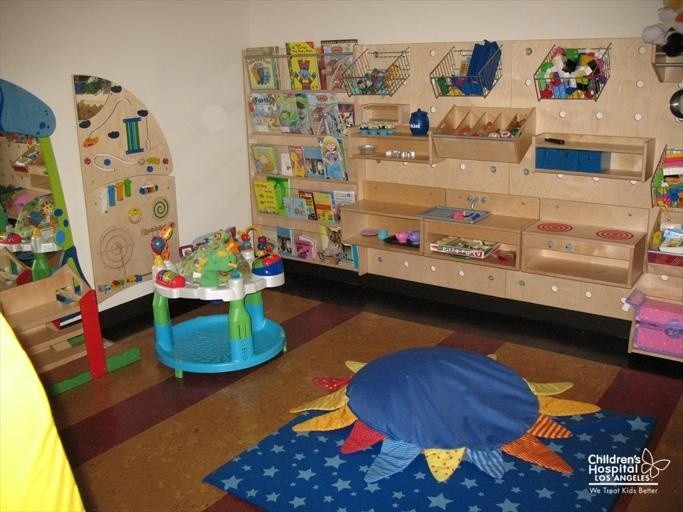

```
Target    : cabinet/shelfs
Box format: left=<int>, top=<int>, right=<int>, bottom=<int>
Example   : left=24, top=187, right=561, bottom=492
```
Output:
left=241, top=37, right=683, bottom=363
left=0, top=264, right=142, bottom=398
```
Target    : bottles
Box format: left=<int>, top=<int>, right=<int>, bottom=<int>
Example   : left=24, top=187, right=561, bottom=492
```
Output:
left=410, top=108, right=429, bottom=136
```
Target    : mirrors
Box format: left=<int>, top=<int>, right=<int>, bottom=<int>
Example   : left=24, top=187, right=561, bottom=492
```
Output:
left=0, top=78, right=80, bottom=291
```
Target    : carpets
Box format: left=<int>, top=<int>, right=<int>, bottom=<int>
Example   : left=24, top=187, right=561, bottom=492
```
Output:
left=200, top=347, right=657, bottom=511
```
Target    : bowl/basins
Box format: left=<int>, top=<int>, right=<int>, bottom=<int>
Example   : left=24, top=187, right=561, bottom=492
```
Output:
left=359, top=146, right=376, bottom=156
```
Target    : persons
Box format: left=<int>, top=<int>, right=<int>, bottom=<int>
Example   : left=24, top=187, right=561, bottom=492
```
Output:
left=149, top=219, right=288, bottom=380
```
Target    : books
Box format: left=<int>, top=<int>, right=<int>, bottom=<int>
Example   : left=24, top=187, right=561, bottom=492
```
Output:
left=650, top=152, right=682, bottom=255
left=242, top=39, right=356, bottom=265
